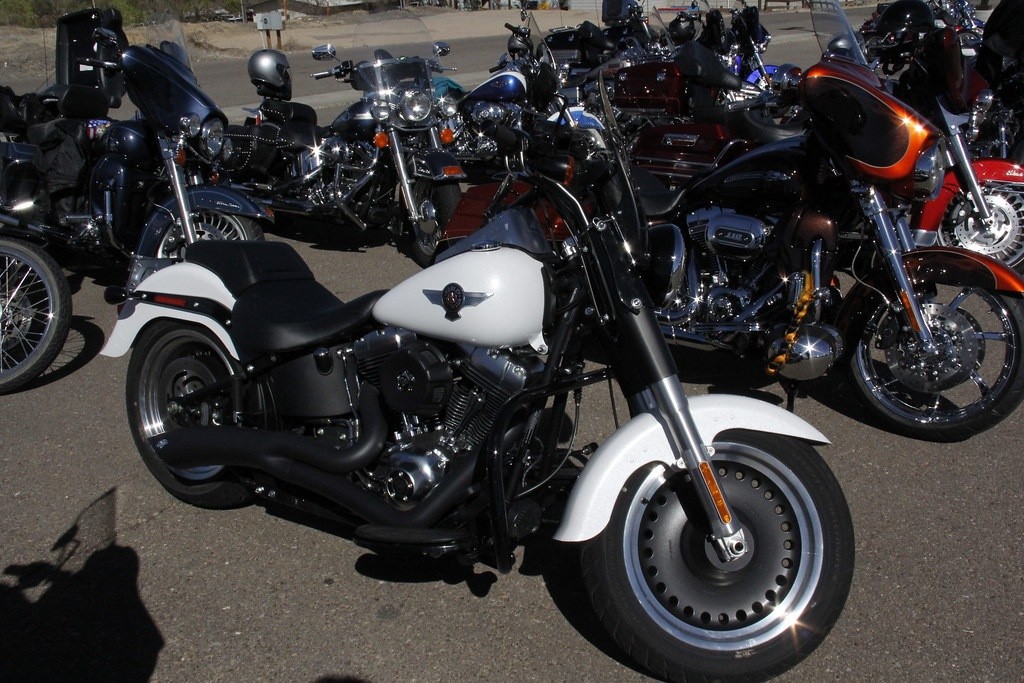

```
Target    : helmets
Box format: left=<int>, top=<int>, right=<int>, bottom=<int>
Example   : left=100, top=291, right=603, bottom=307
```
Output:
left=248, top=49, right=290, bottom=88
left=876, top=0, right=936, bottom=35
left=507, top=35, right=533, bottom=59
left=669, top=12, right=696, bottom=45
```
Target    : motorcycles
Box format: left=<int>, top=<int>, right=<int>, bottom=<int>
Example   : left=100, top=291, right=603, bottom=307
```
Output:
left=0, top=1, right=1024, bottom=683
